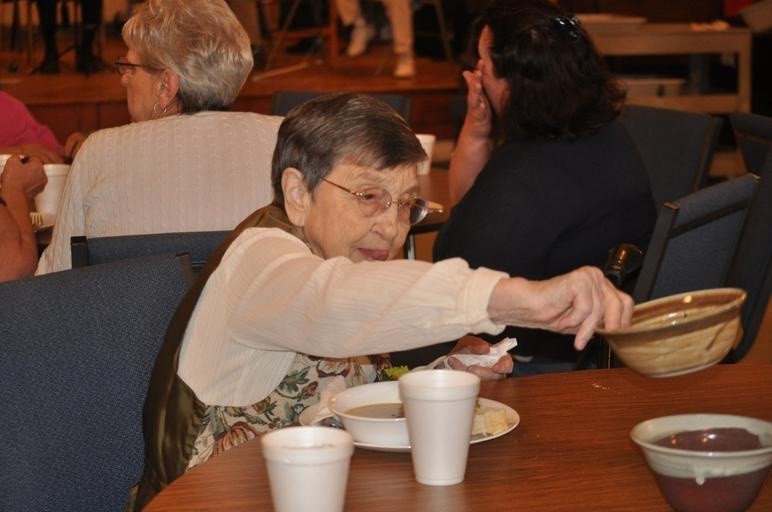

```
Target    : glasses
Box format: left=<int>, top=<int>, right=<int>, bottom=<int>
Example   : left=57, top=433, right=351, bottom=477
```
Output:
left=321, top=178, right=428, bottom=225
left=116, top=57, right=141, bottom=74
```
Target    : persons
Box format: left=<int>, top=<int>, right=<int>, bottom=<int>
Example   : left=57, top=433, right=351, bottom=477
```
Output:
left=1, top=154, right=47, bottom=285
left=0, top=83, right=87, bottom=164
left=428, top=2, right=659, bottom=378
left=132, top=87, right=634, bottom=512
left=33, top=0, right=284, bottom=277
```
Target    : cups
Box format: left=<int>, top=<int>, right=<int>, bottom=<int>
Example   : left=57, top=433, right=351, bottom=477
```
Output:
left=258, top=425, right=354, bottom=512
left=397, top=367, right=482, bottom=489
left=413, top=133, right=435, bottom=176
left=1, top=154, right=25, bottom=174
left=30, top=163, right=68, bottom=213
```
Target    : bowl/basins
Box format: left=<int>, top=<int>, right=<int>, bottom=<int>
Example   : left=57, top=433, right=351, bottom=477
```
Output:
left=332, top=381, right=411, bottom=444
left=597, top=284, right=748, bottom=383
left=628, top=411, right=772, bottom=511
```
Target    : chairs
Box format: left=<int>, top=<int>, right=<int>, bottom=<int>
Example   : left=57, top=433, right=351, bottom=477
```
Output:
left=0, top=23, right=772, bottom=512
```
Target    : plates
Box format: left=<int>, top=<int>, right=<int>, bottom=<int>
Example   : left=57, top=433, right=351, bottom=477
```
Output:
left=297, top=396, right=520, bottom=448
